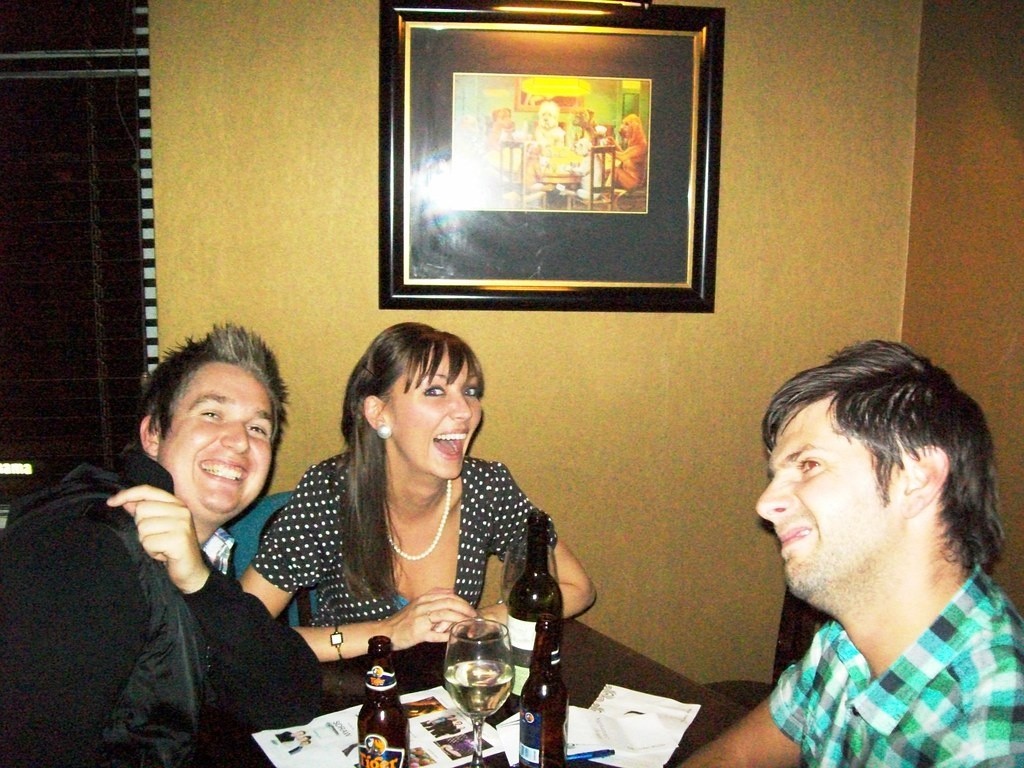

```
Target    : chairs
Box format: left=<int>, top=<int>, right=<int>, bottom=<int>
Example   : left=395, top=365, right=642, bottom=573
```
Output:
left=259, top=504, right=313, bottom=632
left=700, top=583, right=835, bottom=712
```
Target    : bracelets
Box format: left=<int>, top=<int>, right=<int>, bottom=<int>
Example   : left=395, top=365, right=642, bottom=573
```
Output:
left=330, top=625, right=343, bottom=660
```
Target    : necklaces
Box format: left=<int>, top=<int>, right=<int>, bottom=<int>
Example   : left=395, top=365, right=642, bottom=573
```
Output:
left=389, top=480, right=451, bottom=560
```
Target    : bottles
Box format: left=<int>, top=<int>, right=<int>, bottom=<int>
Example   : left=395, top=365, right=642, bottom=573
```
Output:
left=357, top=636, right=410, bottom=768
left=519, top=614, right=570, bottom=768
left=506, top=509, right=564, bottom=714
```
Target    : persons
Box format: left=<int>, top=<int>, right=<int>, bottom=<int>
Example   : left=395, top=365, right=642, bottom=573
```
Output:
left=678, top=339, right=1024, bottom=768
left=238, top=322, right=595, bottom=663
left=276, top=730, right=311, bottom=755
left=421, top=715, right=465, bottom=739
left=0, top=323, right=323, bottom=768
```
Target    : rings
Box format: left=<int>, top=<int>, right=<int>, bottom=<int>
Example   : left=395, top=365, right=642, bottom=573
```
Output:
left=428, top=612, right=434, bottom=624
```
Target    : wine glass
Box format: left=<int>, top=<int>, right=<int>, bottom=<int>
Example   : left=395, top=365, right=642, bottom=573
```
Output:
left=445, top=619, right=516, bottom=768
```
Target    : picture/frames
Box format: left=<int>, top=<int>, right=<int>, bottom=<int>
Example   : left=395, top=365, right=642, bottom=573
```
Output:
left=378, top=0, right=726, bottom=313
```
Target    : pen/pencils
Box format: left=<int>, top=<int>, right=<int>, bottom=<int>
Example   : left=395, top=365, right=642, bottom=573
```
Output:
left=564, top=749, right=616, bottom=764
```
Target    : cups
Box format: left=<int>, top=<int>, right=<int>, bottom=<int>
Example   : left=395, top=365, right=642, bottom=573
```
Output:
left=500, top=540, right=558, bottom=606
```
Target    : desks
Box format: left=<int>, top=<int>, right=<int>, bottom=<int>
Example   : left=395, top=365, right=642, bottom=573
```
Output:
left=198, top=617, right=750, bottom=768
